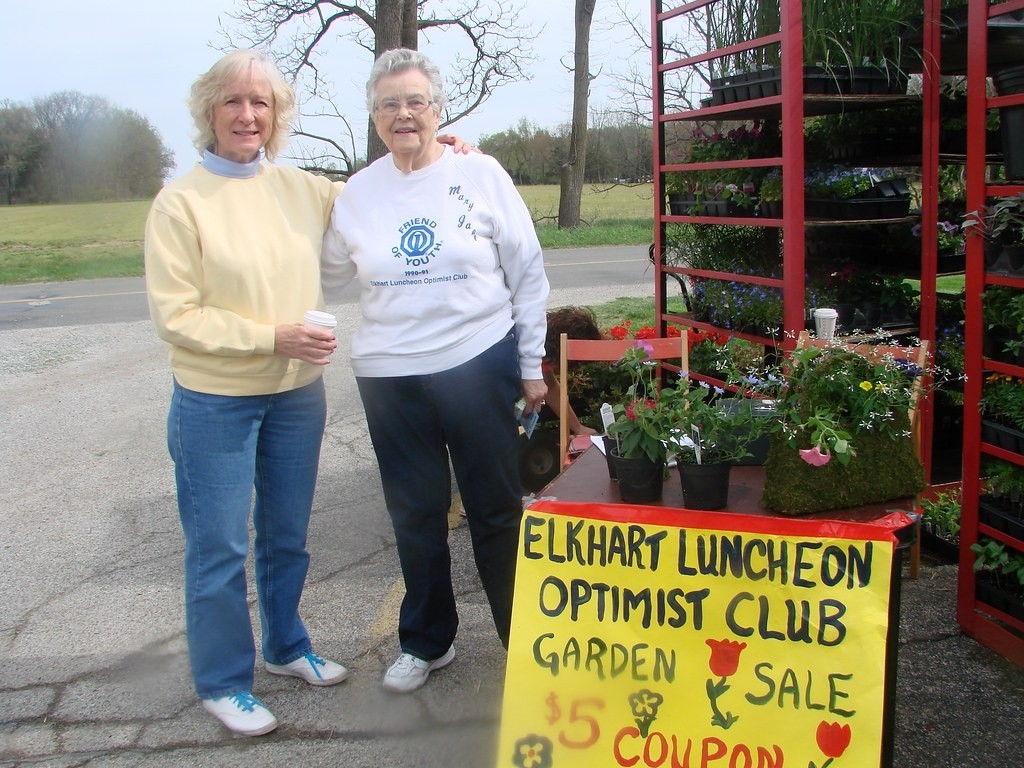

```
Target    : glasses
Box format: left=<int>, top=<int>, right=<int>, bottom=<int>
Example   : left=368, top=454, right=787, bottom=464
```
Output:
left=375, top=97, right=434, bottom=117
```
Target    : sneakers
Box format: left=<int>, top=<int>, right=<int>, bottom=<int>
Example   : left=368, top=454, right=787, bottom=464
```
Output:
left=383, top=642, right=455, bottom=694
left=203, top=690, right=278, bottom=736
left=264, top=649, right=348, bottom=686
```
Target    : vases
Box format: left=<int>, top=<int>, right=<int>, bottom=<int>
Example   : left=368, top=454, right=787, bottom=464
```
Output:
left=893, top=298, right=908, bottom=321
left=838, top=302, right=855, bottom=326
left=707, top=308, right=720, bottom=328
left=941, top=253, right=966, bottom=273
left=994, top=64, right=1024, bottom=181
left=861, top=299, right=880, bottom=328
left=755, top=320, right=770, bottom=338
left=881, top=304, right=892, bottom=322
left=770, top=321, right=783, bottom=341
left=699, top=98, right=712, bottom=107
left=669, top=174, right=912, bottom=219
left=675, top=450, right=731, bottom=511
left=691, top=302, right=706, bottom=323
left=740, top=319, right=755, bottom=335
left=601, top=436, right=664, bottom=503
left=980, top=415, right=1024, bottom=456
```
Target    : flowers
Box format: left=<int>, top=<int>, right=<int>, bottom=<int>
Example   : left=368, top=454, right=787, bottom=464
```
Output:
left=976, top=372, right=1024, bottom=431
left=911, top=215, right=965, bottom=261
left=664, top=120, right=906, bottom=201
left=539, top=266, right=972, bottom=470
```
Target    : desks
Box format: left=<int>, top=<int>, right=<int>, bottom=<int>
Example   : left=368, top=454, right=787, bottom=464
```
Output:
left=523, top=430, right=919, bottom=768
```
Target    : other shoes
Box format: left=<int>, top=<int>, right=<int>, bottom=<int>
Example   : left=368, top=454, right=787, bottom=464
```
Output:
left=459, top=500, right=467, bottom=517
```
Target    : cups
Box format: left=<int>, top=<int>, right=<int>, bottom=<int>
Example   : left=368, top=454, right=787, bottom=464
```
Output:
left=814, top=308, right=838, bottom=341
left=304, top=311, right=338, bottom=358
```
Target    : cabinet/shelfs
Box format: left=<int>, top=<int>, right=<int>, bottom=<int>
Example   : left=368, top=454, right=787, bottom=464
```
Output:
left=950, top=0, right=1024, bottom=674
left=644, top=0, right=982, bottom=518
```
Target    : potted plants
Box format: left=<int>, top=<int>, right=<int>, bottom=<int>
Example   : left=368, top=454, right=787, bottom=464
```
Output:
left=711, top=1, right=964, bottom=106
left=978, top=284, right=1024, bottom=366
left=970, top=538, right=1023, bottom=621
left=960, top=191, right=1024, bottom=270
left=922, top=486, right=961, bottom=564
left=979, top=461, right=1024, bottom=541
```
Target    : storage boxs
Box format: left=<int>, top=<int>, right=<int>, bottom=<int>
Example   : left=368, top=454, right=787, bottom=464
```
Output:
left=715, top=398, right=781, bottom=466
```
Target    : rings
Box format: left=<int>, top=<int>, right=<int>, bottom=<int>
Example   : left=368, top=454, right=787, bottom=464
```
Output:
left=540, top=400, right=545, bottom=405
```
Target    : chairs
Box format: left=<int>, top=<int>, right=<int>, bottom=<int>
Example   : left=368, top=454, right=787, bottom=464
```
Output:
left=559, top=330, right=688, bottom=480
left=793, top=329, right=930, bottom=578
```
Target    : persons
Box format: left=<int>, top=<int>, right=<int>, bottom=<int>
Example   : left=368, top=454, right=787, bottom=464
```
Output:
left=321, top=48, right=548, bottom=693
left=142, top=50, right=484, bottom=735
left=458, top=304, right=599, bottom=518
